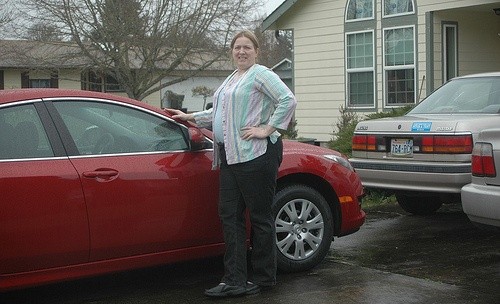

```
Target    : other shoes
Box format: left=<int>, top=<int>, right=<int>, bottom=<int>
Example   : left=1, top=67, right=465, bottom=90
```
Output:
left=204, top=283, right=246, bottom=297
left=245, top=283, right=270, bottom=296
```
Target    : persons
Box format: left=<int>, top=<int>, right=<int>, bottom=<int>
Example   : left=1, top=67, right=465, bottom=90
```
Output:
left=164, top=29, right=297, bottom=297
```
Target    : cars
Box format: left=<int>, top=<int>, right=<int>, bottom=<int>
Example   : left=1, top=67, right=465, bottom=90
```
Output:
left=461, top=129, right=500, bottom=230
left=0, top=89, right=365, bottom=290
left=348, top=71, right=500, bottom=217
